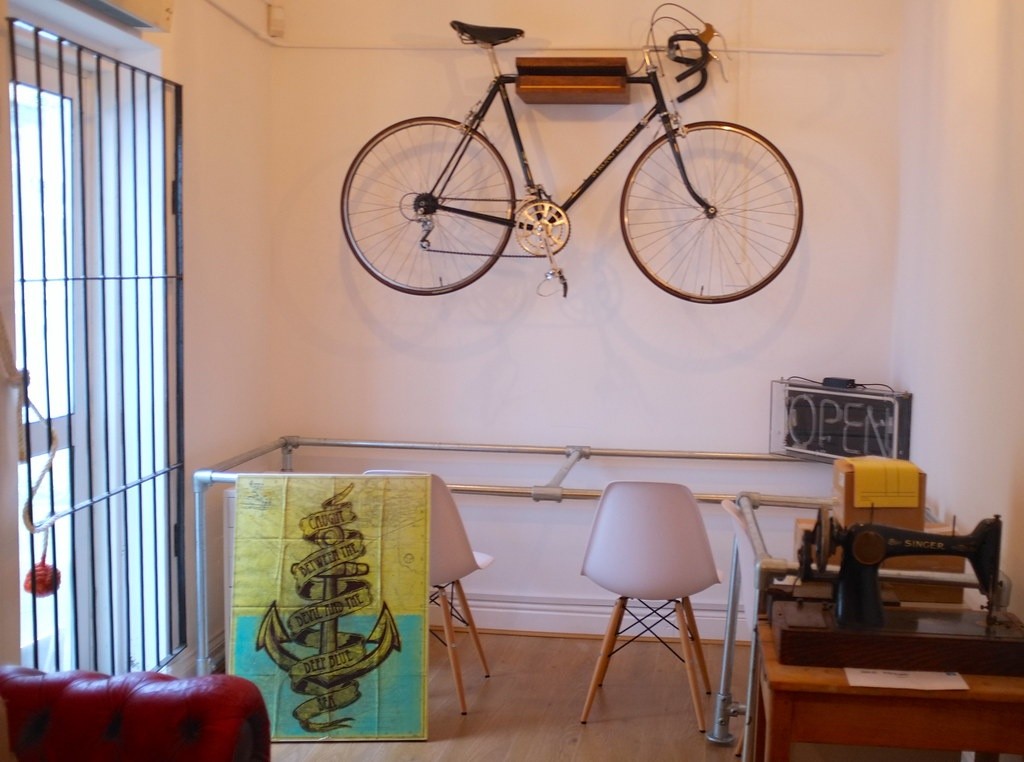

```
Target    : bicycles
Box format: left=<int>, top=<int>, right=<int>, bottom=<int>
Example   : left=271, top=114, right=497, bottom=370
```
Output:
left=338, top=2, right=802, bottom=305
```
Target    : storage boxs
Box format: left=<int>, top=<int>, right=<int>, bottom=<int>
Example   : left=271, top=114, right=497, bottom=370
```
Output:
left=832, top=458, right=926, bottom=535
left=794, top=517, right=967, bottom=604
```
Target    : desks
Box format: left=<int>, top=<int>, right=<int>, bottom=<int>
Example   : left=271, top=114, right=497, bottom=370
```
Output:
left=749, top=622, right=1024, bottom=762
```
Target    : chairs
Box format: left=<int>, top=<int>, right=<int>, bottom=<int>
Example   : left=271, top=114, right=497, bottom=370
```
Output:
left=361, top=470, right=495, bottom=717
left=721, top=498, right=750, bottom=759
left=580, top=479, right=723, bottom=734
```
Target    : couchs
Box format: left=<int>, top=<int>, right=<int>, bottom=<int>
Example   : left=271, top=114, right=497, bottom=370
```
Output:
left=0, top=665, right=272, bottom=762
